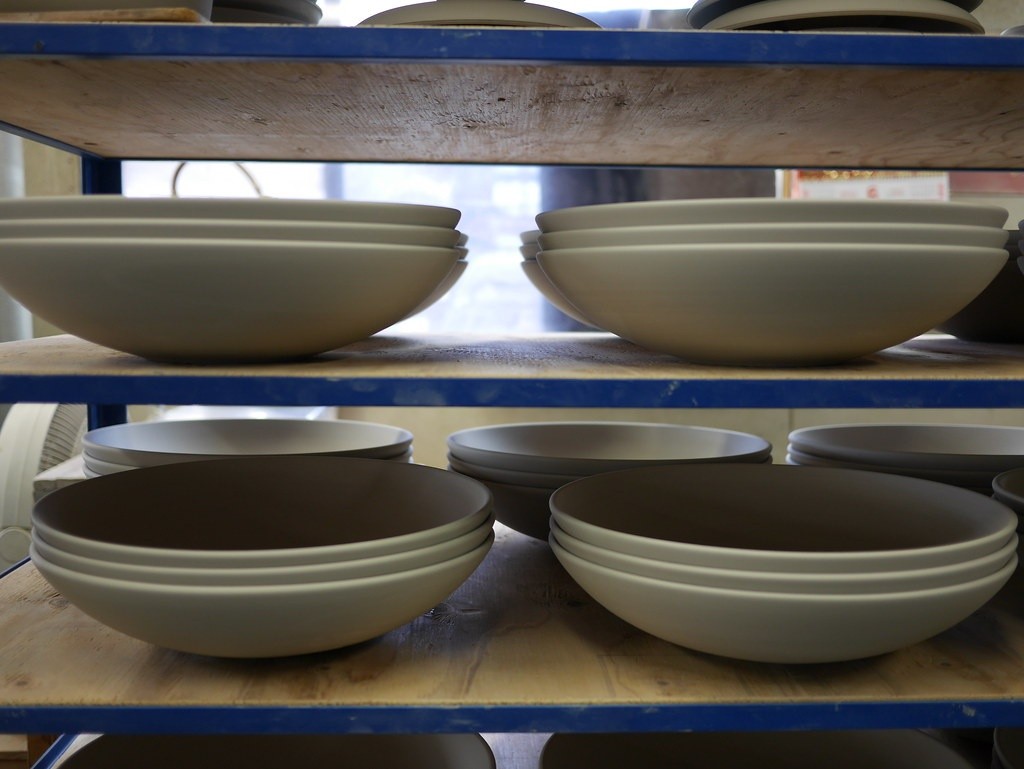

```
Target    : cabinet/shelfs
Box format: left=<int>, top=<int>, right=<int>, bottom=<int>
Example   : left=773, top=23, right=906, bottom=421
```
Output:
left=0, top=0, right=1024, bottom=769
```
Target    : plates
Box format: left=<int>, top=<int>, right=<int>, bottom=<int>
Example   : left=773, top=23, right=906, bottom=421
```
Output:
left=349, top=0, right=988, bottom=34
left=521, top=197, right=1024, bottom=364
left=0, top=195, right=469, bottom=362
left=31, top=419, right=1024, bottom=666
